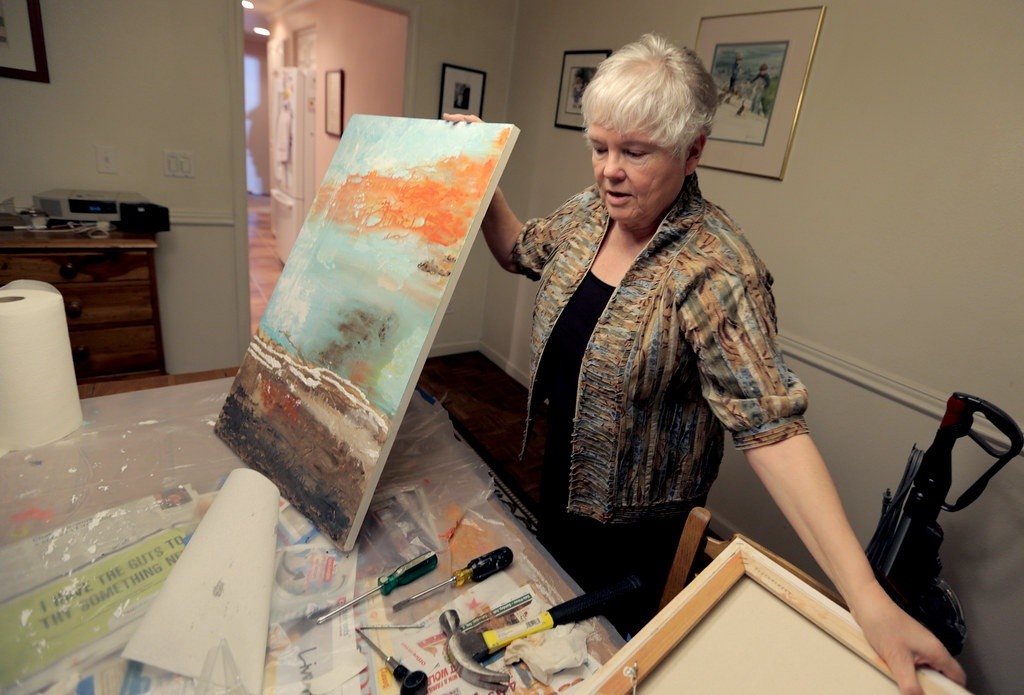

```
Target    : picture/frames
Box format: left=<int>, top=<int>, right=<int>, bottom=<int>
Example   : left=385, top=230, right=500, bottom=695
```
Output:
left=0, top=0, right=52, bottom=84
left=690, top=4, right=827, bottom=181
left=325, top=68, right=346, bottom=138
left=438, top=62, right=487, bottom=122
left=570, top=537, right=975, bottom=695
left=554, top=48, right=612, bottom=132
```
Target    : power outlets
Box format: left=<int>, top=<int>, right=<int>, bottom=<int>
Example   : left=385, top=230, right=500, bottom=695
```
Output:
left=95, top=145, right=115, bottom=173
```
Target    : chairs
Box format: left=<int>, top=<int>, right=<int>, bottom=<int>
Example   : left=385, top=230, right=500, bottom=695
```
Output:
left=657, top=505, right=849, bottom=612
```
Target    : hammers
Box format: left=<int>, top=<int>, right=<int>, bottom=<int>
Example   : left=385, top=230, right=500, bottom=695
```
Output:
left=439, top=572, right=642, bottom=692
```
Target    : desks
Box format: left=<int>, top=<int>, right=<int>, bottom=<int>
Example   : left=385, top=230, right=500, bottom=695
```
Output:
left=0, top=378, right=625, bottom=695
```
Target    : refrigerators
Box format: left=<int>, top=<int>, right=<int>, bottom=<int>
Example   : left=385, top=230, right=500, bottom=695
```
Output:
left=269, top=68, right=314, bottom=264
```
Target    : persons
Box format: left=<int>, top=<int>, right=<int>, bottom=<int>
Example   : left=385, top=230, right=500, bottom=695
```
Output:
left=440, top=35, right=968, bottom=695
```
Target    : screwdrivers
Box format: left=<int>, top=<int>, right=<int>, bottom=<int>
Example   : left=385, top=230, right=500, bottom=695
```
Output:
left=391, top=545, right=514, bottom=613
left=355, top=628, right=428, bottom=695
left=316, top=550, right=439, bottom=626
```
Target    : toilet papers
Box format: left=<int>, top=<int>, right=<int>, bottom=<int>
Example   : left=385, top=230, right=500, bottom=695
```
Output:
left=1, top=279, right=85, bottom=457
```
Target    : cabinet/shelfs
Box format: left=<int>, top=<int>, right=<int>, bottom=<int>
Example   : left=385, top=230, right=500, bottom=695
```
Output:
left=0, top=229, right=169, bottom=385
